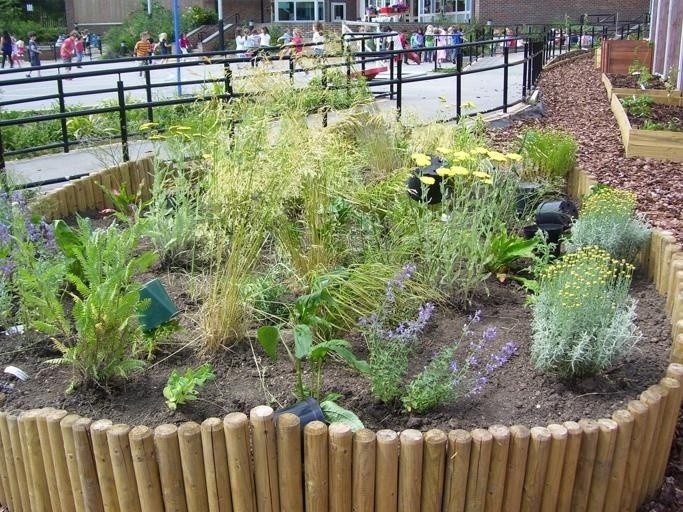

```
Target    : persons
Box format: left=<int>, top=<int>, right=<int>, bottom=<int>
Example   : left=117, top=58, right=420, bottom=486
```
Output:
left=133, top=30, right=193, bottom=77
left=383, top=24, right=468, bottom=67
left=235, top=22, right=325, bottom=76
left=1, top=29, right=102, bottom=80
left=490, top=28, right=594, bottom=56
